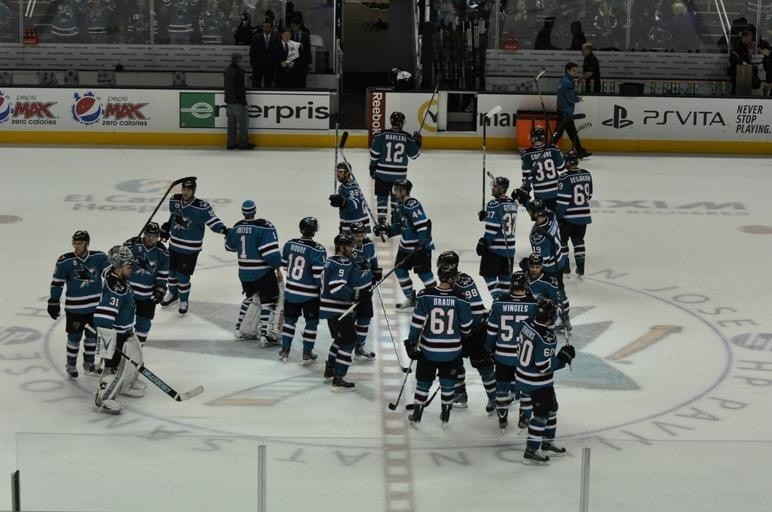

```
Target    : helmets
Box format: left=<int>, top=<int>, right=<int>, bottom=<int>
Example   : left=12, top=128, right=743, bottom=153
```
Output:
left=336, top=161, right=351, bottom=172
left=143, top=222, right=160, bottom=236
left=529, top=250, right=544, bottom=268
left=333, top=231, right=357, bottom=249
left=562, top=150, right=580, bottom=159
left=389, top=109, right=406, bottom=127
left=529, top=125, right=548, bottom=141
left=71, top=229, right=90, bottom=241
left=528, top=198, right=544, bottom=213
left=393, top=177, right=414, bottom=193
left=438, top=265, right=458, bottom=284
left=299, top=214, right=318, bottom=235
left=436, top=249, right=459, bottom=268
left=535, top=300, right=561, bottom=325
left=182, top=179, right=196, bottom=191
left=493, top=176, right=511, bottom=192
left=349, top=219, right=365, bottom=233
left=510, top=271, right=527, bottom=288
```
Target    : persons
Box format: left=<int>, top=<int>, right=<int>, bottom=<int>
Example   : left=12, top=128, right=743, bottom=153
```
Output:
left=555, top=150, right=593, bottom=279
left=549, top=62, right=593, bottom=161
left=406, top=252, right=575, bottom=467
left=277, top=217, right=327, bottom=364
left=320, top=222, right=377, bottom=393
left=577, top=43, right=601, bottom=93
left=249, top=9, right=311, bottom=90
left=512, top=189, right=574, bottom=336
left=224, top=200, right=283, bottom=348
left=474, top=176, right=518, bottom=300
left=48, top=229, right=111, bottom=381
left=519, top=127, right=566, bottom=212
left=329, top=162, right=372, bottom=240
left=728, top=30, right=755, bottom=95
left=758, top=41, right=772, bottom=97
left=382, top=178, right=435, bottom=313
left=154, top=2, right=252, bottom=44
left=94, top=223, right=169, bottom=416
left=224, top=52, right=257, bottom=150
left=161, top=179, right=228, bottom=317
left=630, top=3, right=747, bottom=51
left=419, top=8, right=444, bottom=89
left=369, top=111, right=422, bottom=235
left=48, top=0, right=150, bottom=43
left=499, top=2, right=626, bottom=51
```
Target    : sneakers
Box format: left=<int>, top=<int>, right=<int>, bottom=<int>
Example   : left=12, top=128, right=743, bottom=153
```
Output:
left=329, top=373, right=357, bottom=393
left=178, top=299, right=190, bottom=314
left=518, top=418, right=531, bottom=429
left=159, top=289, right=176, bottom=307
left=484, top=397, right=498, bottom=412
left=522, top=446, right=551, bottom=461
left=495, top=407, right=509, bottom=419
left=563, top=266, right=569, bottom=273
left=397, top=295, right=417, bottom=307
left=438, top=404, right=455, bottom=421
left=107, top=244, right=136, bottom=269
left=407, top=404, right=423, bottom=420
left=575, top=266, right=584, bottom=274
left=64, top=360, right=79, bottom=379
left=85, top=360, right=103, bottom=374
left=302, top=345, right=319, bottom=360
left=356, top=343, right=374, bottom=357
left=540, top=438, right=567, bottom=455
left=453, top=392, right=468, bottom=403
left=323, top=359, right=339, bottom=377
left=277, top=345, right=290, bottom=358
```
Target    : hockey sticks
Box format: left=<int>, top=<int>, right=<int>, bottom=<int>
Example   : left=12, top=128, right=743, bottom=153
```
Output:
left=84, top=323, right=204, bottom=403
left=526, top=113, right=586, bottom=182
left=332, top=109, right=343, bottom=195
left=481, top=104, right=503, bottom=214
left=338, top=130, right=388, bottom=244
left=374, top=279, right=413, bottom=375
left=487, top=169, right=516, bottom=278
left=534, top=67, right=554, bottom=137
left=388, top=347, right=414, bottom=411
left=335, top=238, right=425, bottom=323
left=418, top=72, right=447, bottom=135
left=405, top=386, right=442, bottom=411
left=138, top=176, right=198, bottom=240
left=536, top=226, right=577, bottom=373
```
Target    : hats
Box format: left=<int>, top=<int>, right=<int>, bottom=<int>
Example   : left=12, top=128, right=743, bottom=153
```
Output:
left=241, top=200, right=257, bottom=216
